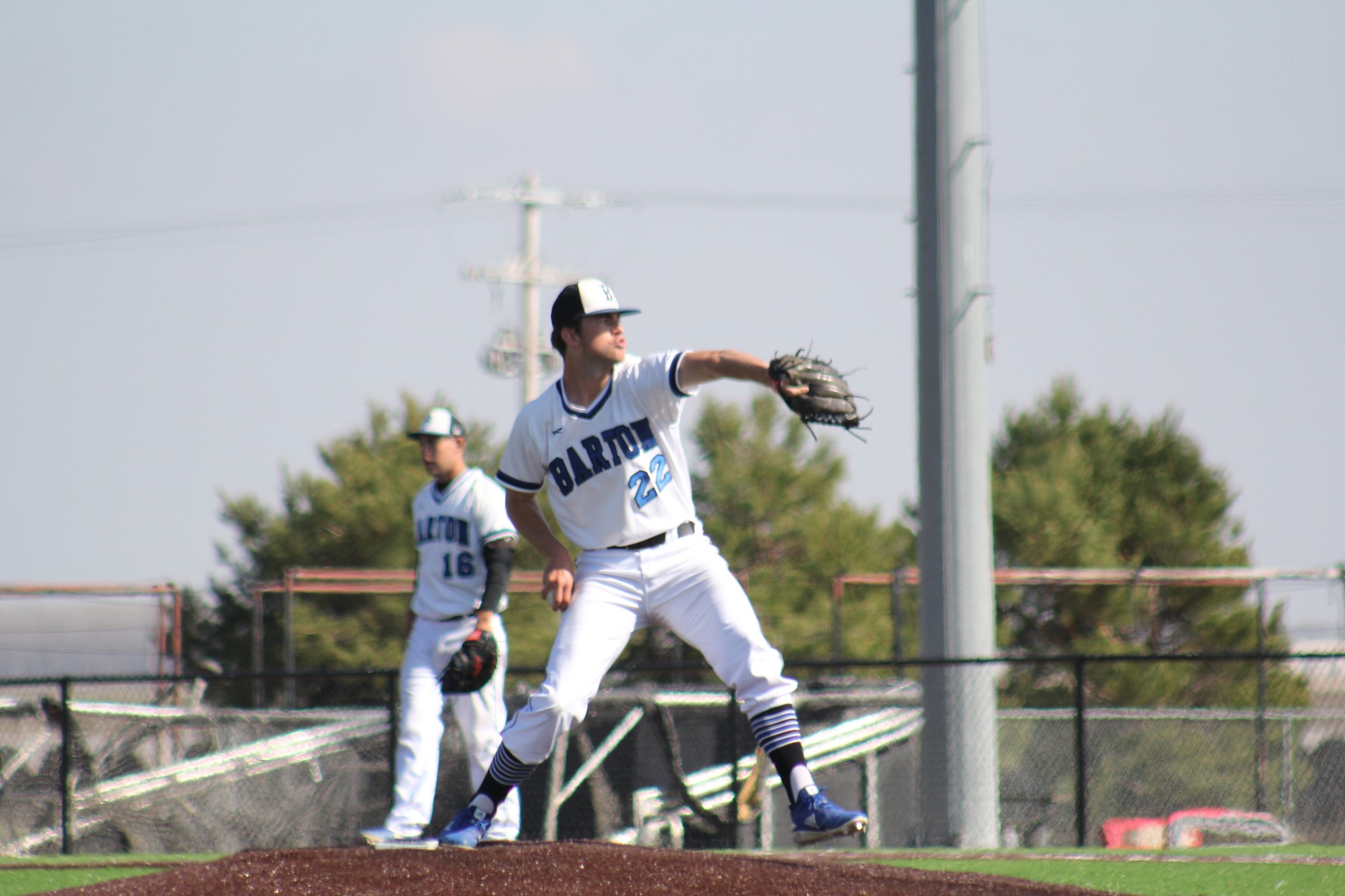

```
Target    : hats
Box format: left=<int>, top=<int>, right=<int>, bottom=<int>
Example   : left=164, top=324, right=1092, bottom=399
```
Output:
left=551, top=280, right=639, bottom=326
left=404, top=406, right=467, bottom=440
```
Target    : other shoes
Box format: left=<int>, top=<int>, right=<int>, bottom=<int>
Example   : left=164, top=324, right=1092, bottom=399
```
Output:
left=362, top=828, right=436, bottom=850
left=481, top=819, right=519, bottom=843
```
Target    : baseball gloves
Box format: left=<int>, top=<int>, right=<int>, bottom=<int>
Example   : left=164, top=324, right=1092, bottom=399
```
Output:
left=441, top=629, right=498, bottom=694
left=769, top=356, right=860, bottom=427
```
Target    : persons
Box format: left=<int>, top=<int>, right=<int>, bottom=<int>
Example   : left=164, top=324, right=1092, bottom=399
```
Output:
left=359, top=409, right=521, bottom=842
left=438, top=278, right=869, bottom=848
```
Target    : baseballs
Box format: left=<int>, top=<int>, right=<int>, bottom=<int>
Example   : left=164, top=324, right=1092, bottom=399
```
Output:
left=545, top=589, right=555, bottom=605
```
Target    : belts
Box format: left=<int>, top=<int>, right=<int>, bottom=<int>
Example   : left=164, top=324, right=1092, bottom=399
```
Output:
left=606, top=522, right=693, bottom=549
left=441, top=610, right=474, bottom=621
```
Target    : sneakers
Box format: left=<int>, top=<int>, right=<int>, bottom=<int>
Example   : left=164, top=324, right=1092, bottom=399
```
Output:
left=788, top=784, right=868, bottom=843
left=438, top=807, right=490, bottom=849
